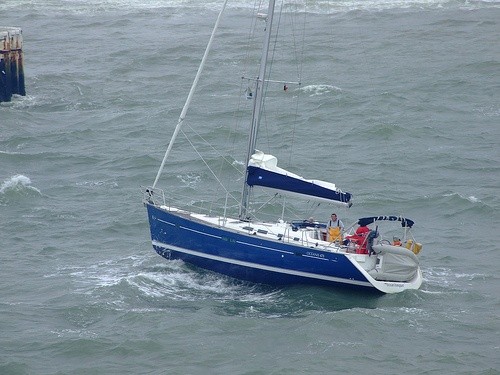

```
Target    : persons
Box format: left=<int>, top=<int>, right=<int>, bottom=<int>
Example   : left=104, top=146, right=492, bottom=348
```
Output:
left=326, top=213, right=344, bottom=244
left=345, top=224, right=372, bottom=254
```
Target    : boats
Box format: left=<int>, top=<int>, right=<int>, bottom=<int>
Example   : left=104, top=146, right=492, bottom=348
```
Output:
left=144, top=0, right=425, bottom=295
